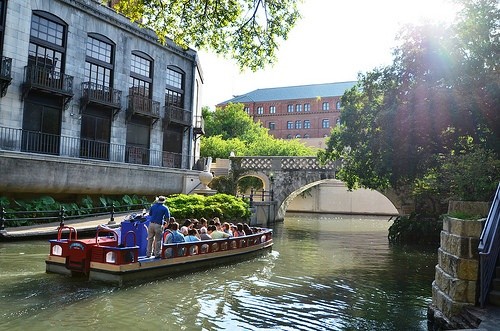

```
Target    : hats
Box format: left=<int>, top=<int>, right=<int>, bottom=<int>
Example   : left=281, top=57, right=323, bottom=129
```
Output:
left=157, top=196, right=166, bottom=203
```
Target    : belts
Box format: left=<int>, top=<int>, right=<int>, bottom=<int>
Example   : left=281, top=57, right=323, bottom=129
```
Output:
left=151, top=221, right=159, bottom=224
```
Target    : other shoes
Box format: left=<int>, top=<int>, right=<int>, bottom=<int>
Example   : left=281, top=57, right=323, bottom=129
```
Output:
left=147, top=256, right=150, bottom=258
left=155, top=256, right=160, bottom=259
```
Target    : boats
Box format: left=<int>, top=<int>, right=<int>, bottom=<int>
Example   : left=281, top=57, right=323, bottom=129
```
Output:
left=44, top=217, right=274, bottom=286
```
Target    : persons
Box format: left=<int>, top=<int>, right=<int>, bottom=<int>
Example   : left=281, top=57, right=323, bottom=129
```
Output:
left=161, top=217, right=265, bottom=258
left=147, top=196, right=170, bottom=259
